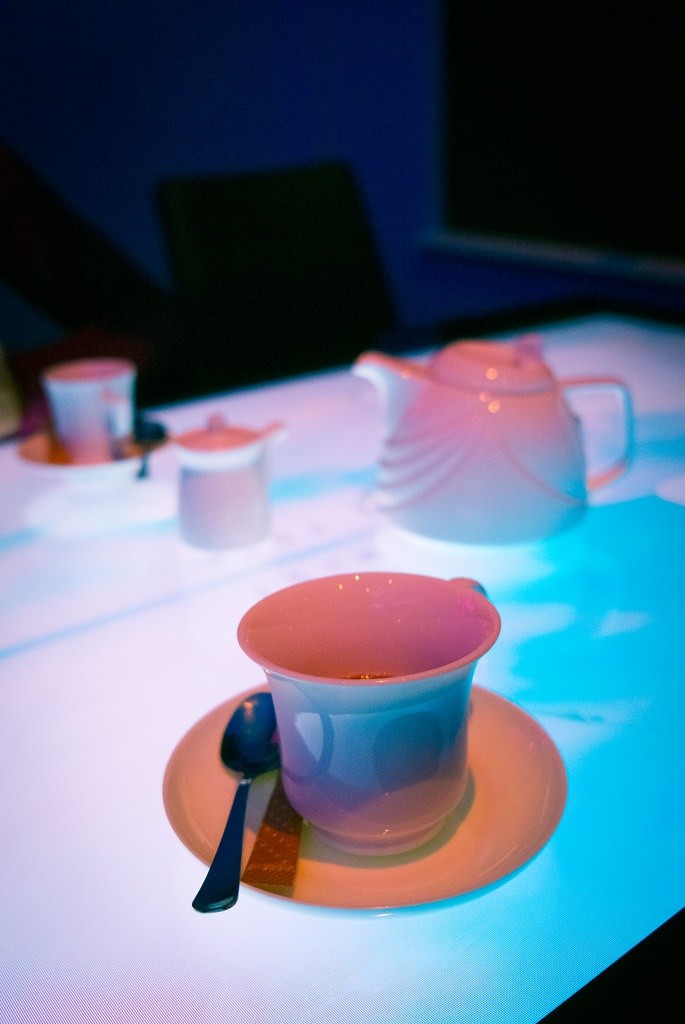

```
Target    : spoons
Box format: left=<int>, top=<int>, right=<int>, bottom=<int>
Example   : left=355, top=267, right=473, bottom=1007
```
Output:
left=192, top=691, right=282, bottom=913
left=130, top=421, right=166, bottom=477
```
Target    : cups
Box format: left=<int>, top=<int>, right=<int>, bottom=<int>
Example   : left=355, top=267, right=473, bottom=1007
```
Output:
left=43, top=357, right=137, bottom=464
left=236, top=572, right=501, bottom=857
left=168, top=416, right=283, bottom=547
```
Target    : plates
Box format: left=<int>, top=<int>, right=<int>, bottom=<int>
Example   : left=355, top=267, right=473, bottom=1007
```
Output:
left=21, top=431, right=169, bottom=477
left=161, top=683, right=568, bottom=908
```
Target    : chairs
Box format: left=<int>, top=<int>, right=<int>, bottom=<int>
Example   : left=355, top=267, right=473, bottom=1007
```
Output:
left=0, top=144, right=417, bottom=423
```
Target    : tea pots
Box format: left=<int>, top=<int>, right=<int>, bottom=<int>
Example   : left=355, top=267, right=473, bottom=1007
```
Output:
left=350, top=337, right=636, bottom=547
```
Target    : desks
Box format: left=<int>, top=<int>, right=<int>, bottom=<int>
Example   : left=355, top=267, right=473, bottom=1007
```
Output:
left=0, top=293, right=684, bottom=1024
